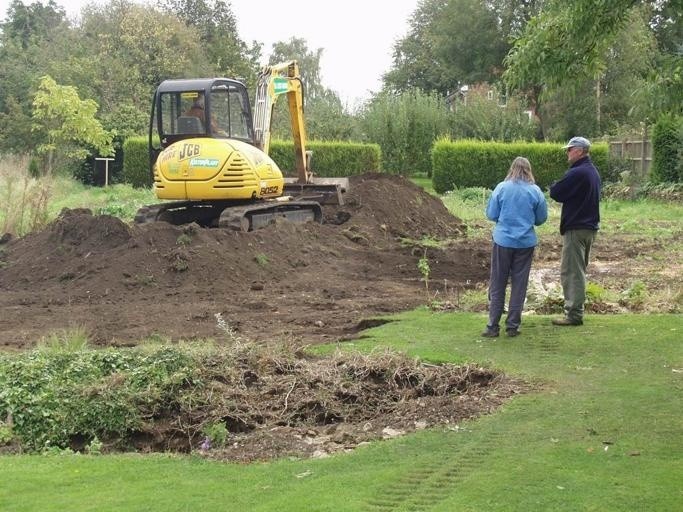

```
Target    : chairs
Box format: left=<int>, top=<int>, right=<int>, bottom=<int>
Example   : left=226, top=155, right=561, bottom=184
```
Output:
left=176, top=116, right=205, bottom=134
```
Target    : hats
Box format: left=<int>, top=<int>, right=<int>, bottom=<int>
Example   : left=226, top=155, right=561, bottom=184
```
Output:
left=561, top=137, right=589, bottom=149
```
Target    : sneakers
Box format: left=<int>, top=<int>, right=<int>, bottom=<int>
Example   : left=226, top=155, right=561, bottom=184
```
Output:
left=550, top=319, right=572, bottom=325
left=481, top=331, right=498, bottom=337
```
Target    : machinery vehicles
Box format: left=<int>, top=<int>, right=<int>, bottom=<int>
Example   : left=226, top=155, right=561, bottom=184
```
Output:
left=132, top=58, right=349, bottom=230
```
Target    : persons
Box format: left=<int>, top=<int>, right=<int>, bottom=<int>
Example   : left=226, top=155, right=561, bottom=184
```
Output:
left=481, top=157, right=548, bottom=337
left=550, top=136, right=601, bottom=325
left=187, top=95, right=217, bottom=133
